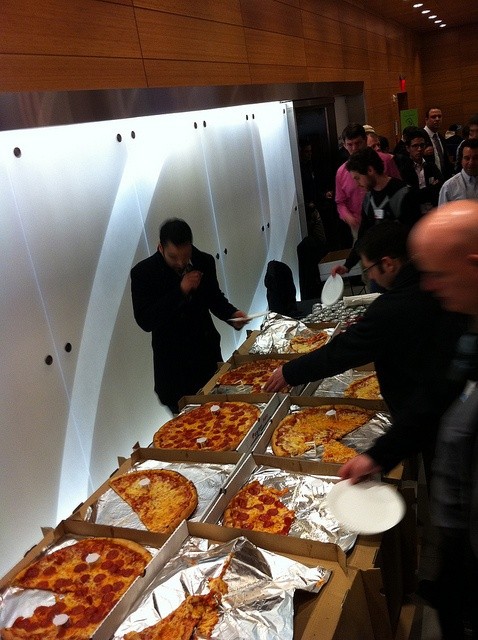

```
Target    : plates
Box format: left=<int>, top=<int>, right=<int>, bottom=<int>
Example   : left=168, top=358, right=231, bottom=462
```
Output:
left=321, top=273, right=344, bottom=305
left=227, top=311, right=270, bottom=322
left=327, top=479, right=407, bottom=535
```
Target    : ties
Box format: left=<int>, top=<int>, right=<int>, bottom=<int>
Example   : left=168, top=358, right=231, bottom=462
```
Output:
left=433, top=134, right=443, bottom=164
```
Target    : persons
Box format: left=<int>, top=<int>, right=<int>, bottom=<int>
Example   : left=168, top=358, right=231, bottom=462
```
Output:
left=130, top=216, right=249, bottom=417
left=366, top=131, right=381, bottom=153
left=403, top=130, right=444, bottom=222
left=331, top=146, right=422, bottom=278
left=437, top=137, right=478, bottom=207
left=333, top=122, right=404, bottom=248
left=422, top=105, right=446, bottom=179
left=444, top=124, right=458, bottom=139
left=454, top=127, right=470, bottom=169
left=338, top=199, right=478, bottom=639
left=393, top=125, right=413, bottom=153
left=467, top=117, right=478, bottom=139
left=299, top=136, right=333, bottom=243
left=263, top=221, right=465, bottom=422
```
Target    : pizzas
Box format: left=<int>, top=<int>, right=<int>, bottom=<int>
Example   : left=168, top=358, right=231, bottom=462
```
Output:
left=0, top=537, right=154, bottom=640
left=216, top=359, right=292, bottom=394
left=153, top=400, right=263, bottom=452
left=124, top=574, right=229, bottom=640
left=109, top=468, right=199, bottom=535
left=289, top=326, right=331, bottom=355
left=219, top=478, right=296, bottom=537
left=345, top=372, right=385, bottom=401
left=271, top=405, right=376, bottom=464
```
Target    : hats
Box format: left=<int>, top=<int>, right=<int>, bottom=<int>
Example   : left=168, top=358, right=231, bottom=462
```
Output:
left=362, top=125, right=375, bottom=134
left=449, top=124, right=461, bottom=131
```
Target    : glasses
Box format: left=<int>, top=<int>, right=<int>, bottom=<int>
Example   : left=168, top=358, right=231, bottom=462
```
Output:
left=410, top=143, right=426, bottom=148
left=362, top=255, right=393, bottom=276
